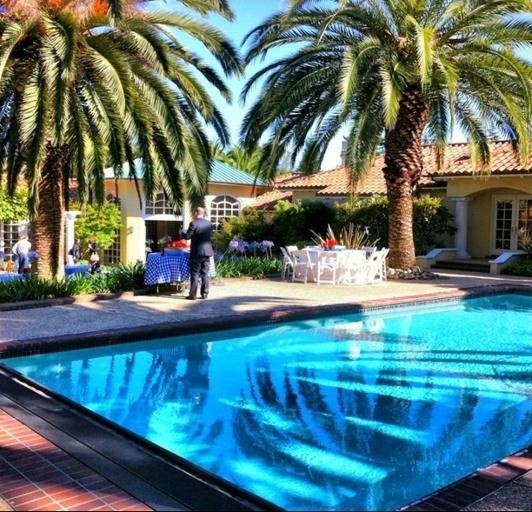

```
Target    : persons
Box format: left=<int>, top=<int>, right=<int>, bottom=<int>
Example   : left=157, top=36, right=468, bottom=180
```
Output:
left=11, top=234, right=32, bottom=274
left=86, top=237, right=97, bottom=265
left=72, top=238, right=81, bottom=265
left=180, top=207, right=214, bottom=300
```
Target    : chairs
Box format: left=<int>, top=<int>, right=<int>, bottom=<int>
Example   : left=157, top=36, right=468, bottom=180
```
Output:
left=279, top=245, right=389, bottom=287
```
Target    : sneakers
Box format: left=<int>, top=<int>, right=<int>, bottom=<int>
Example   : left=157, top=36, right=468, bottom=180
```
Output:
left=184, top=293, right=208, bottom=301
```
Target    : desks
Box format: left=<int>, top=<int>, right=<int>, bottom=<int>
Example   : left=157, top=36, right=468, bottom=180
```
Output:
left=148, top=252, right=215, bottom=296
